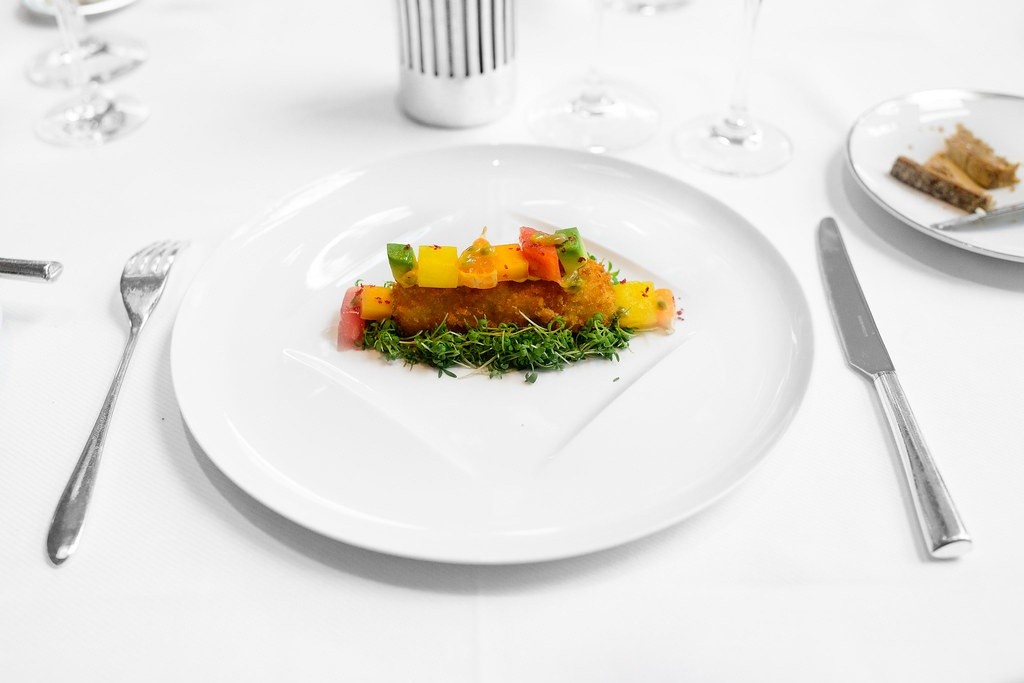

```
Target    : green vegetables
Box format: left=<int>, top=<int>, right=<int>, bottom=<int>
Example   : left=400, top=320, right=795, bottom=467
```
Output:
left=352, top=252, right=639, bottom=383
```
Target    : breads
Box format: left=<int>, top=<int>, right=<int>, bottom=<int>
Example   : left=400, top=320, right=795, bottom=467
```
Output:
left=889, top=125, right=1021, bottom=214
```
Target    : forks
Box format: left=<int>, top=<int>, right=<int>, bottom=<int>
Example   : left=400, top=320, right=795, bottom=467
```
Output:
left=46, top=239, right=189, bottom=565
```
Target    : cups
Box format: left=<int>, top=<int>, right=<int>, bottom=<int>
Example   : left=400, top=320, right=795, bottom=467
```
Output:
left=394, top=0, right=519, bottom=126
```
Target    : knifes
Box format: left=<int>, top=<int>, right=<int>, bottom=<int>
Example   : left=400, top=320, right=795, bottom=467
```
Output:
left=930, top=203, right=1024, bottom=231
left=817, top=215, right=972, bottom=561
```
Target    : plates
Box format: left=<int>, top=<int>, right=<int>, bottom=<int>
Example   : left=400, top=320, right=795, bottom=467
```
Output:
left=169, top=141, right=817, bottom=566
left=846, top=89, right=1024, bottom=265
